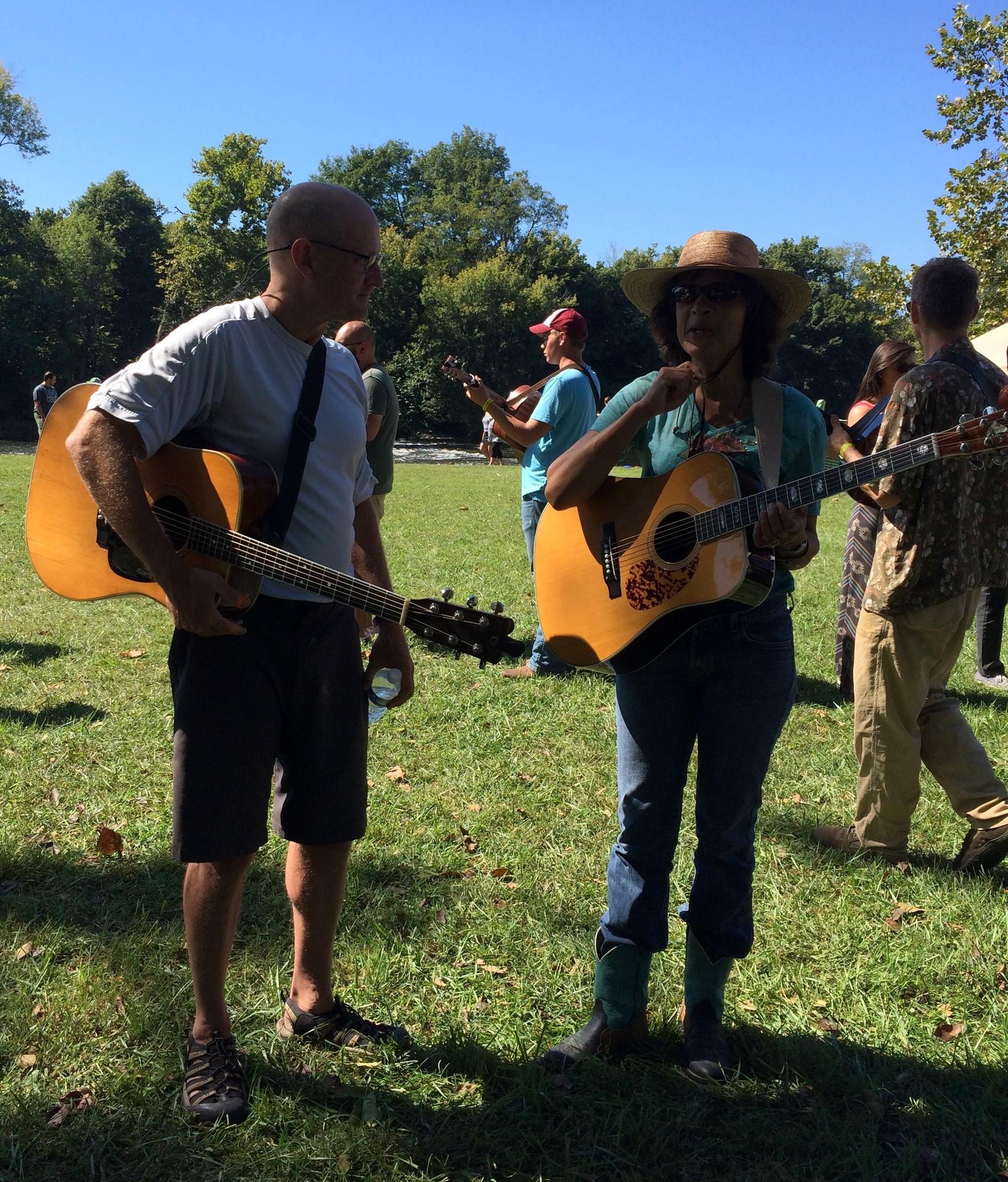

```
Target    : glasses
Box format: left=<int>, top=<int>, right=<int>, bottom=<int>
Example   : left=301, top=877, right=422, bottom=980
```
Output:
left=304, top=237, right=383, bottom=273
left=889, top=361, right=915, bottom=373
left=665, top=283, right=754, bottom=306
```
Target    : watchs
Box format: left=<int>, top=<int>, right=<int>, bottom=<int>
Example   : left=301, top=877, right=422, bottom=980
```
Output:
left=777, top=535, right=809, bottom=559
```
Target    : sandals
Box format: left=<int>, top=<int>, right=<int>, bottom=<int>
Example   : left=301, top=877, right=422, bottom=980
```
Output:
left=275, top=995, right=411, bottom=1057
left=182, top=1036, right=249, bottom=1127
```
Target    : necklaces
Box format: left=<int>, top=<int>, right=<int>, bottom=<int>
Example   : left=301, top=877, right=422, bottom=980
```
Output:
left=728, top=390, right=744, bottom=425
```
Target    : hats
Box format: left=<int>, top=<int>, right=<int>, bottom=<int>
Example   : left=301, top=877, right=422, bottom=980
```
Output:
left=529, top=308, right=590, bottom=342
left=622, top=231, right=811, bottom=330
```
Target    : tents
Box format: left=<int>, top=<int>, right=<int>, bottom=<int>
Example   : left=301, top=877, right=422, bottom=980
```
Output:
left=970, top=323, right=1008, bottom=376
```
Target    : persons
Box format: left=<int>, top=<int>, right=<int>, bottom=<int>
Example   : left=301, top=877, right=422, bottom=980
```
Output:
left=833, top=340, right=918, bottom=700
left=33, top=372, right=57, bottom=437
left=541, top=233, right=825, bottom=1083
left=810, top=256, right=1008, bottom=876
left=65, top=182, right=418, bottom=1124
left=465, top=309, right=612, bottom=678
left=335, top=321, right=399, bottom=660
left=975, top=585, right=1008, bottom=691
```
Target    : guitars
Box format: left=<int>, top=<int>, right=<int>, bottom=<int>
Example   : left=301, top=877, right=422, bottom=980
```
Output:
left=440, top=354, right=543, bottom=453
left=24, top=380, right=525, bottom=673
left=818, top=406, right=883, bottom=510
left=532, top=407, right=1008, bottom=676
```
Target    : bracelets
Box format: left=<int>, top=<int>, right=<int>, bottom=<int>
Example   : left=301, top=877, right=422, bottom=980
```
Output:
left=482, top=398, right=494, bottom=411
left=41, top=416, right=45, bottom=418
left=839, top=443, right=856, bottom=460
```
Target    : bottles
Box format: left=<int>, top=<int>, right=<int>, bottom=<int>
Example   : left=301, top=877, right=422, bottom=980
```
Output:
left=368, top=667, right=402, bottom=723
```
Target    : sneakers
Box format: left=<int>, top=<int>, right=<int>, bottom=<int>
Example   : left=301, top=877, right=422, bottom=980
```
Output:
left=952, top=823, right=1007, bottom=874
left=809, top=823, right=908, bottom=864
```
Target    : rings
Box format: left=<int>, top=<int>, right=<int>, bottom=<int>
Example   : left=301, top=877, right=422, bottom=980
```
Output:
left=768, top=540, right=780, bottom=548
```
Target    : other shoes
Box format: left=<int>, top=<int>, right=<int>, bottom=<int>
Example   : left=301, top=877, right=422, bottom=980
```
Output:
left=502, top=660, right=538, bottom=676
left=973, top=668, right=1008, bottom=691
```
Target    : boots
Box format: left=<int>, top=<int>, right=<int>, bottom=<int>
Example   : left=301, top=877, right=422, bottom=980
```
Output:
left=682, top=928, right=732, bottom=1083
left=542, top=928, right=649, bottom=1066
